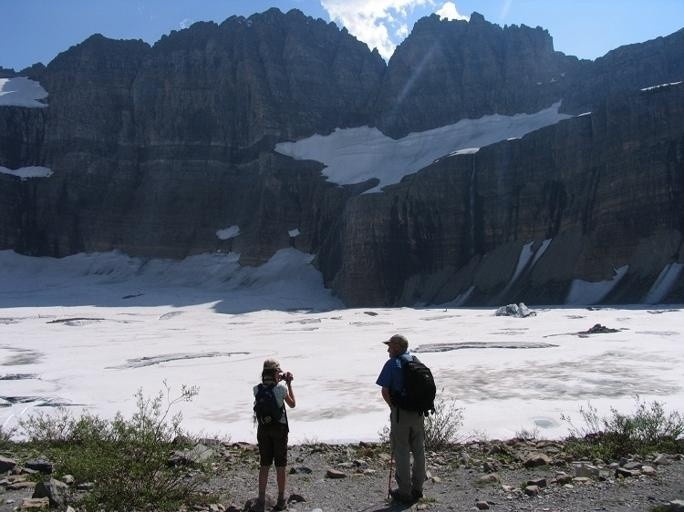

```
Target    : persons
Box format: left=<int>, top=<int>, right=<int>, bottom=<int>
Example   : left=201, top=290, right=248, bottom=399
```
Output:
left=254, top=359, right=295, bottom=509
left=376, top=334, right=425, bottom=500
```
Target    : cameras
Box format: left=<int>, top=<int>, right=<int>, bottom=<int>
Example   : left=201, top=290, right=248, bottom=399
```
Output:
left=279, top=373, right=287, bottom=381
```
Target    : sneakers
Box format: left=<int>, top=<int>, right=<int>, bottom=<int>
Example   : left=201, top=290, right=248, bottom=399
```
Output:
left=254, top=498, right=288, bottom=511
left=388, top=488, right=422, bottom=502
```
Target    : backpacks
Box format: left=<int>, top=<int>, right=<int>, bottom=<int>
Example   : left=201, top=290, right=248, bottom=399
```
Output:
left=392, top=356, right=436, bottom=411
left=253, top=384, right=282, bottom=424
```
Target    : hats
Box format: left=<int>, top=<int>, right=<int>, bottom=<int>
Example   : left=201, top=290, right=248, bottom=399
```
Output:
left=262, top=359, right=282, bottom=372
left=382, top=333, right=408, bottom=348
